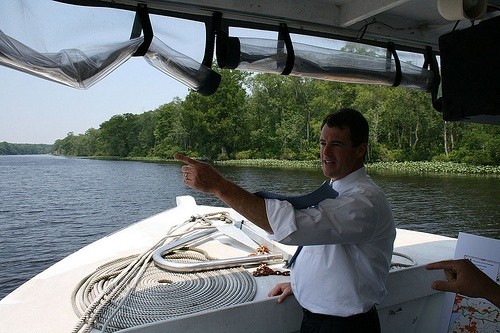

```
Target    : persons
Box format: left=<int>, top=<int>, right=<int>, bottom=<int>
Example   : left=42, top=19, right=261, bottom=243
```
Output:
left=173, top=109, right=396, bottom=333
left=425, top=259, right=500, bottom=309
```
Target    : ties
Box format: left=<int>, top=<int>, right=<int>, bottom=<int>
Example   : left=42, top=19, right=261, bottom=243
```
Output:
left=286, top=181, right=332, bottom=269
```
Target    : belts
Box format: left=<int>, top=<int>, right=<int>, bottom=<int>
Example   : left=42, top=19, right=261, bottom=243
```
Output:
left=301, top=305, right=378, bottom=322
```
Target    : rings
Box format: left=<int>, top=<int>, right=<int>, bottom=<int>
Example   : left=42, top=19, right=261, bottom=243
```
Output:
left=185, top=173, right=189, bottom=180
